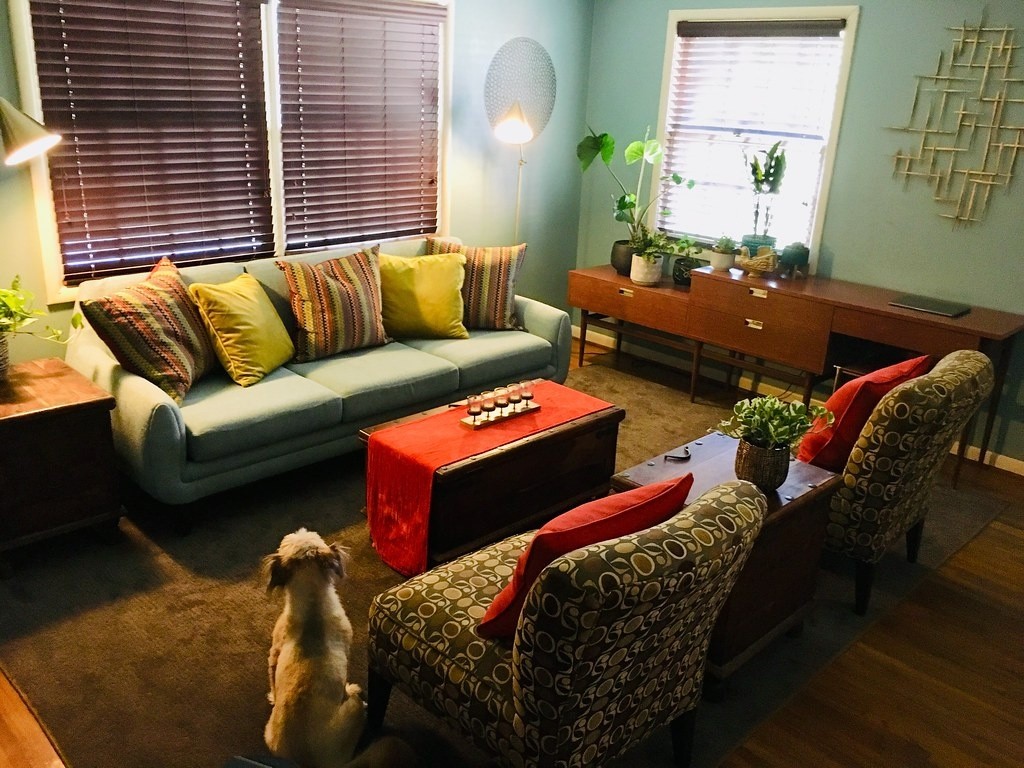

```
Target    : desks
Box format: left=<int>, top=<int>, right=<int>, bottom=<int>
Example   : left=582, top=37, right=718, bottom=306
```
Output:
left=358, top=378, right=625, bottom=560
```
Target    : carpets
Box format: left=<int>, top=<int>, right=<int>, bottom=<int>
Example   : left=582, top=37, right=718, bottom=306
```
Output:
left=0, top=355, right=1013, bottom=768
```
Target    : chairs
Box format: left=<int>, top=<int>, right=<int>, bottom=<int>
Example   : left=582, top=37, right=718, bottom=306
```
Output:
left=732, top=350, right=995, bottom=615
left=365, top=480, right=767, bottom=768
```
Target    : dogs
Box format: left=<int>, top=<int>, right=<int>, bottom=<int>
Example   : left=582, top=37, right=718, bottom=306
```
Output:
left=262, top=526, right=367, bottom=768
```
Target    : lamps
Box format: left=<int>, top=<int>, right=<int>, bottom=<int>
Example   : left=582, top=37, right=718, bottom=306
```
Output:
left=493, top=101, right=533, bottom=247
left=0, top=96, right=63, bottom=166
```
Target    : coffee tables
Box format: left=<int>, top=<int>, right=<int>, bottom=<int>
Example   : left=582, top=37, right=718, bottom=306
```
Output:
left=609, top=431, right=843, bottom=692
left=0, top=357, right=116, bottom=526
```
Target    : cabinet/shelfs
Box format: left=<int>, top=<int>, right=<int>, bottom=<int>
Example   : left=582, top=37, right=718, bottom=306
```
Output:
left=569, top=265, right=1024, bottom=489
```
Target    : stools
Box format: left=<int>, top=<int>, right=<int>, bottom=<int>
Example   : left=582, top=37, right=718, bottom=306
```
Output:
left=831, top=354, right=888, bottom=395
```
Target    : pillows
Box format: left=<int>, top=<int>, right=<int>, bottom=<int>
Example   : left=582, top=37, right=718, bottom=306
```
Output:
left=362, top=248, right=469, bottom=338
left=797, top=354, right=932, bottom=466
left=426, top=237, right=527, bottom=329
left=188, top=273, right=294, bottom=387
left=477, top=474, right=693, bottom=643
left=272, top=243, right=395, bottom=363
left=78, top=256, right=218, bottom=407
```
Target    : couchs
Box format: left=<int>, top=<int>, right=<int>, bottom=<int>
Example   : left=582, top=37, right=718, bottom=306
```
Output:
left=66, top=236, right=572, bottom=503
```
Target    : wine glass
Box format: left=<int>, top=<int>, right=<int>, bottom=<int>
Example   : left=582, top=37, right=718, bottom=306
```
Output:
left=480, top=391, right=496, bottom=422
left=494, top=388, right=510, bottom=420
left=507, top=384, right=522, bottom=415
left=467, top=395, right=482, bottom=425
left=520, top=380, right=534, bottom=411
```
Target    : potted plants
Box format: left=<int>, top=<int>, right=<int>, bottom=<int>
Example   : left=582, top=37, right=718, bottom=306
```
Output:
left=706, top=393, right=836, bottom=492
left=711, top=236, right=737, bottom=272
left=625, top=226, right=675, bottom=287
left=575, top=121, right=695, bottom=276
left=781, top=241, right=809, bottom=273
left=0, top=273, right=84, bottom=382
left=742, top=141, right=786, bottom=257
left=673, top=236, right=702, bottom=285
